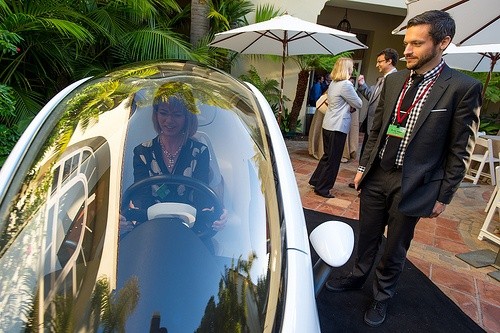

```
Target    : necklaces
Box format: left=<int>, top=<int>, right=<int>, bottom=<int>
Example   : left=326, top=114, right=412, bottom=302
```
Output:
left=161, top=143, right=184, bottom=168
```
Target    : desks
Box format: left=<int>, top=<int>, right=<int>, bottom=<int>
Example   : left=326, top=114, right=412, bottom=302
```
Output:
left=474, top=135, right=500, bottom=183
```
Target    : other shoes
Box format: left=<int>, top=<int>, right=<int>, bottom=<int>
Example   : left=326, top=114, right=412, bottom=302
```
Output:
left=341, top=157, right=348, bottom=163
left=317, top=192, right=335, bottom=198
left=309, top=180, right=317, bottom=188
left=349, top=183, right=355, bottom=188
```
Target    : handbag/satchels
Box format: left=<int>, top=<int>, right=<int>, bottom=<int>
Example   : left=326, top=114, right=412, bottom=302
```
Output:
left=316, top=90, right=330, bottom=115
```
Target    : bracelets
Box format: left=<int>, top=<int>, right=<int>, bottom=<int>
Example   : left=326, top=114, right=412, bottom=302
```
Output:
left=437, top=201, right=445, bottom=206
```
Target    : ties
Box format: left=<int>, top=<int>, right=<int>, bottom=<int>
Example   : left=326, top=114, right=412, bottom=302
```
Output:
left=380, top=73, right=424, bottom=170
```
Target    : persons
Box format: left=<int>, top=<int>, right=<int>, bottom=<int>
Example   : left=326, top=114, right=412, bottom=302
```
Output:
left=349, top=48, right=399, bottom=188
left=321, top=74, right=331, bottom=94
left=325, top=9, right=483, bottom=326
left=309, top=57, right=362, bottom=198
left=130, top=82, right=228, bottom=255
left=310, top=76, right=324, bottom=106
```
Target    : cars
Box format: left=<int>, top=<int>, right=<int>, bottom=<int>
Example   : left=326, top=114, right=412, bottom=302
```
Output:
left=0, top=56, right=357, bottom=333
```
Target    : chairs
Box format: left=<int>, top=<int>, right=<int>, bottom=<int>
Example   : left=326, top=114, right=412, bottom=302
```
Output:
left=477, top=164, right=500, bottom=249
left=465, top=135, right=500, bottom=186
left=194, top=126, right=225, bottom=188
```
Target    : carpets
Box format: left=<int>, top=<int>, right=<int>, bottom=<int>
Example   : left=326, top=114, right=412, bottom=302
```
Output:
left=301, top=206, right=486, bottom=333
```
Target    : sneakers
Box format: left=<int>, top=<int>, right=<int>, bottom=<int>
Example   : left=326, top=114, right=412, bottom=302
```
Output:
left=325, top=274, right=365, bottom=291
left=364, top=299, right=388, bottom=326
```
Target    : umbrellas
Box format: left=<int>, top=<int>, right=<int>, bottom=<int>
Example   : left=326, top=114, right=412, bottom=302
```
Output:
left=208, top=13, right=369, bottom=126
left=392, top=0, right=500, bottom=98
